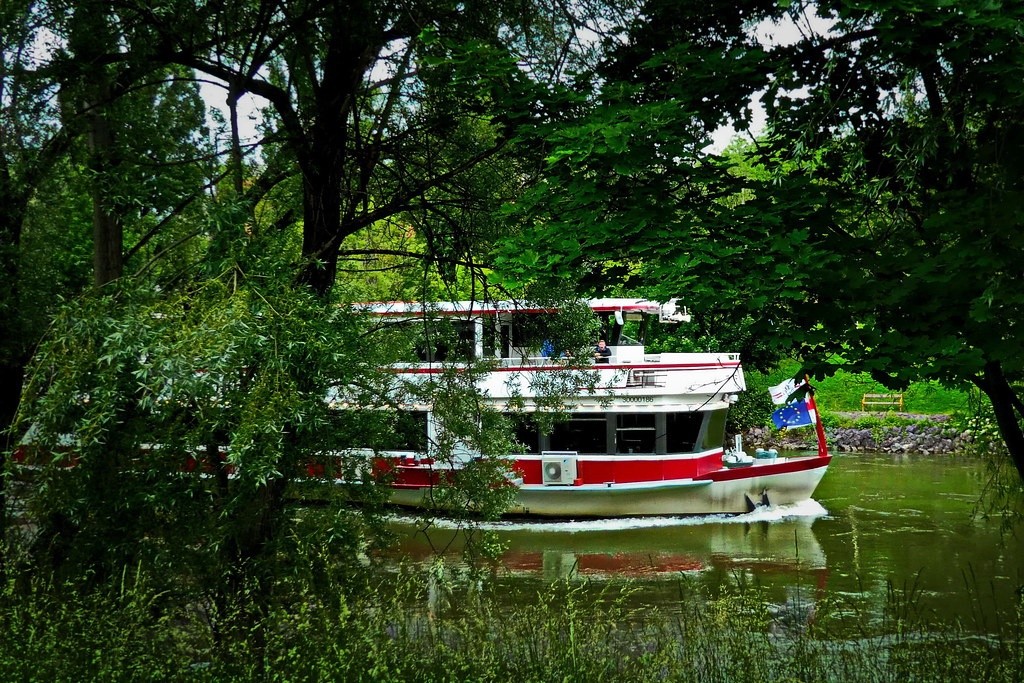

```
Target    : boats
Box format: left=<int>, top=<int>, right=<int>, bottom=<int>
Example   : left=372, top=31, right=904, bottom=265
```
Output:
left=0, top=297, right=835, bottom=524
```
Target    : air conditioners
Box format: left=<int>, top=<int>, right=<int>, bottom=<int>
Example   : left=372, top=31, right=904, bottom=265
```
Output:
left=541, top=458, right=577, bottom=485
left=340, top=450, right=372, bottom=480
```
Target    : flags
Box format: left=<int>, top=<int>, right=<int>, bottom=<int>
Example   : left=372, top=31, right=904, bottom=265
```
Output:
left=770, top=397, right=812, bottom=431
left=768, top=375, right=811, bottom=404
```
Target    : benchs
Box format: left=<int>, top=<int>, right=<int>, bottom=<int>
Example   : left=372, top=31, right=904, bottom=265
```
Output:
left=861, top=393, right=903, bottom=411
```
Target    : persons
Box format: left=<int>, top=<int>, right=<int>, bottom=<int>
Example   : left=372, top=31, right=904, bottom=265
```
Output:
left=542, top=333, right=572, bottom=357
left=591, top=339, right=611, bottom=364
